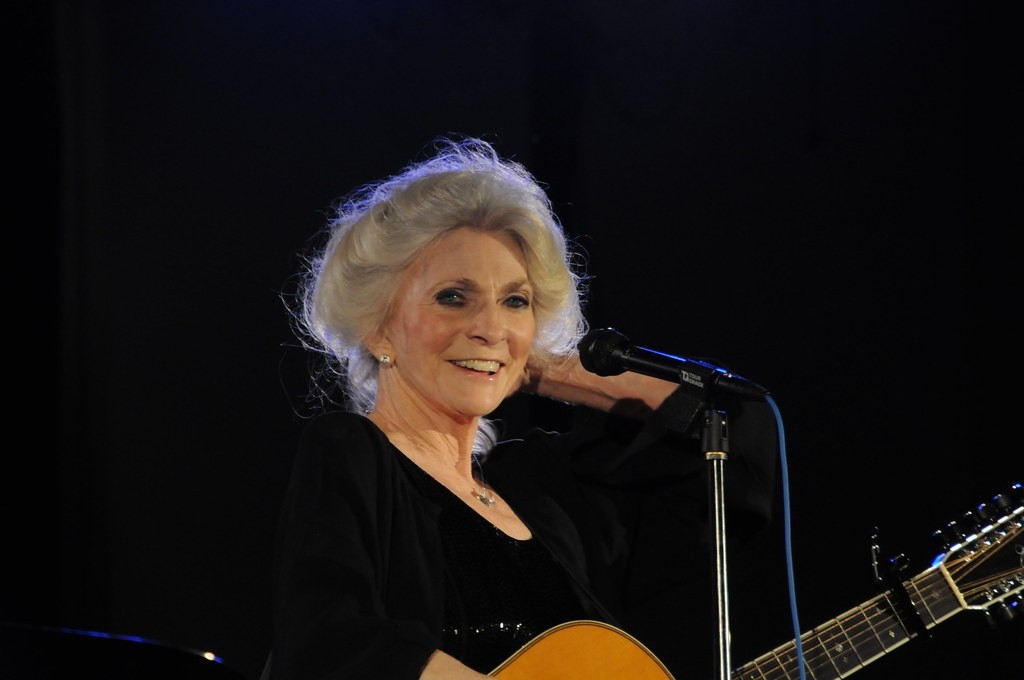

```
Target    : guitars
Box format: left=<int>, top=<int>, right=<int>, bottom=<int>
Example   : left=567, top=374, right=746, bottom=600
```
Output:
left=485, top=480, right=1024, bottom=680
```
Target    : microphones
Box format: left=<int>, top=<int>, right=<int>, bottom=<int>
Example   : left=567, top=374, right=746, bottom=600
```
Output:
left=576, top=327, right=768, bottom=403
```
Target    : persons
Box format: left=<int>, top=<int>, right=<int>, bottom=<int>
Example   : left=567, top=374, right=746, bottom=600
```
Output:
left=261, top=129, right=779, bottom=680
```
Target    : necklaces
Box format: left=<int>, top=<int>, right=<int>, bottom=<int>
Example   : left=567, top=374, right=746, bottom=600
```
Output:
left=366, top=403, right=499, bottom=512
